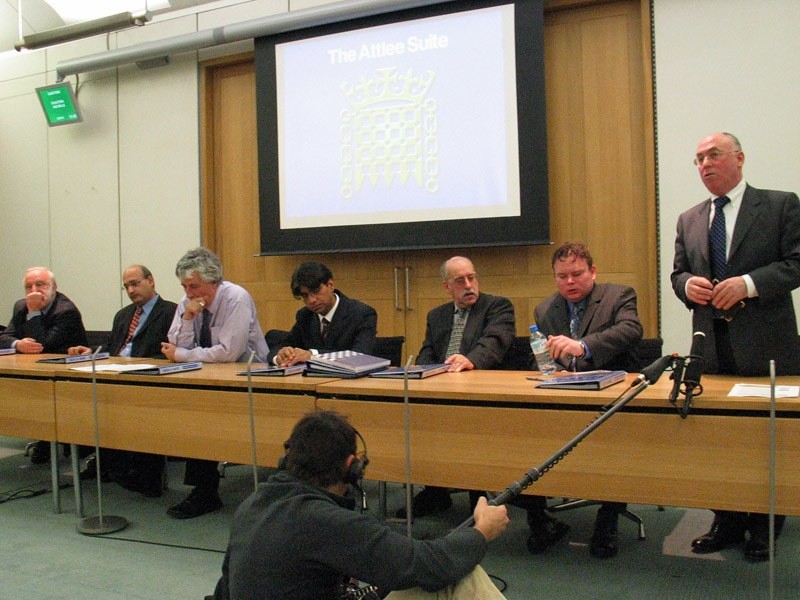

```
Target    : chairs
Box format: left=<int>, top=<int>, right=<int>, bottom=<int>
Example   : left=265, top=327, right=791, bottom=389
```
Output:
left=23, top=331, right=665, bottom=538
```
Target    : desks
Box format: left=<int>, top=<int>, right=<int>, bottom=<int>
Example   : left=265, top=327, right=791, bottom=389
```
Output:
left=0, top=350, right=800, bottom=522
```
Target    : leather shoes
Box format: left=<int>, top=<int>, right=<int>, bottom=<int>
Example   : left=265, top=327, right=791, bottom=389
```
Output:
left=526, top=511, right=571, bottom=555
left=746, top=528, right=777, bottom=560
left=395, top=489, right=454, bottom=520
left=591, top=507, right=620, bottom=556
left=167, top=488, right=221, bottom=518
left=692, top=513, right=744, bottom=553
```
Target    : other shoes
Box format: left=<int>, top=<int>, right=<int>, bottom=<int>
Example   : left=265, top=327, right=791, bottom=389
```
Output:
left=32, top=442, right=53, bottom=464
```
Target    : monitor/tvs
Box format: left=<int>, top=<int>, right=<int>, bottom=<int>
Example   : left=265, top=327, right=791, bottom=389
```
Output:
left=35, top=82, right=84, bottom=127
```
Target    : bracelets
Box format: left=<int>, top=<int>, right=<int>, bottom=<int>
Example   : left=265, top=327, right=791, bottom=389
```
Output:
left=577, top=340, right=585, bottom=359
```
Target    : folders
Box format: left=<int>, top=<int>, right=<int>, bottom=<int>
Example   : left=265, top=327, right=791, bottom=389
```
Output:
left=120, top=361, right=203, bottom=376
left=535, top=371, right=626, bottom=390
left=35, top=352, right=110, bottom=364
left=369, top=364, right=450, bottom=380
left=302, top=349, right=390, bottom=378
left=236, top=363, right=308, bottom=377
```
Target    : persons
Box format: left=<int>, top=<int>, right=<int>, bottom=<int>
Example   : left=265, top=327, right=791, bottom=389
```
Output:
left=203, top=410, right=510, bottom=600
left=670, top=132, right=800, bottom=563
left=67, top=264, right=178, bottom=499
left=526, top=242, right=645, bottom=559
left=266, top=261, right=378, bottom=369
left=395, top=255, right=523, bottom=533
left=0, top=266, right=89, bottom=465
left=162, top=247, right=272, bottom=520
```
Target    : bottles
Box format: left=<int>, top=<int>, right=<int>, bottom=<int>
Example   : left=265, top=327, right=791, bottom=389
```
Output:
left=530, top=325, right=556, bottom=375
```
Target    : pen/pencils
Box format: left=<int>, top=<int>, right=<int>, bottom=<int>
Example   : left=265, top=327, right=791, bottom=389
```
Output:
left=199, top=302, right=204, bottom=306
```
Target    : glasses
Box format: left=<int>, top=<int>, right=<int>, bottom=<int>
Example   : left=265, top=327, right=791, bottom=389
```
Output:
left=694, top=150, right=738, bottom=165
left=455, top=275, right=479, bottom=287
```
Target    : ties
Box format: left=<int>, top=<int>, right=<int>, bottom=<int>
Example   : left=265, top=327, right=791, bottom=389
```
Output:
left=445, top=309, right=466, bottom=359
left=200, top=309, right=212, bottom=348
left=116, top=305, right=144, bottom=355
left=570, top=305, right=583, bottom=339
left=321, top=318, right=329, bottom=336
left=709, top=197, right=731, bottom=319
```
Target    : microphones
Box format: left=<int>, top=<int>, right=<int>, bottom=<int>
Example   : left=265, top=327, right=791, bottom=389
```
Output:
left=682, top=331, right=706, bottom=414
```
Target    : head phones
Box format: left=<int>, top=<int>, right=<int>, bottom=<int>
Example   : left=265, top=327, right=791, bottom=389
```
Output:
left=279, top=423, right=369, bottom=484
